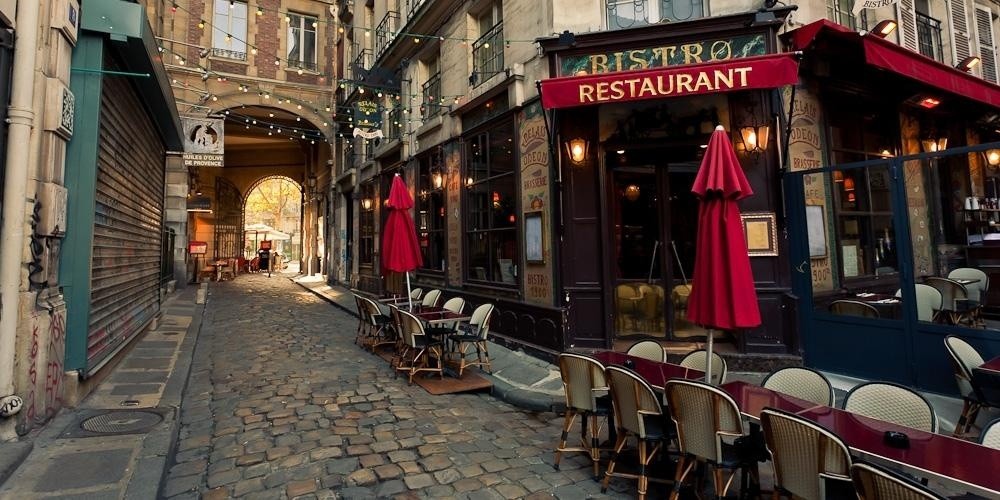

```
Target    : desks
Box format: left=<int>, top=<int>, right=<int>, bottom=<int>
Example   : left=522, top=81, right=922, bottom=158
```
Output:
left=971, top=353, right=1000, bottom=409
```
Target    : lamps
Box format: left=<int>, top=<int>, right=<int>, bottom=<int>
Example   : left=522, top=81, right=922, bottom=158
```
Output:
left=833, top=171, right=857, bottom=202
left=872, top=20, right=897, bottom=35
left=360, top=192, right=373, bottom=211
left=921, top=116, right=949, bottom=161
left=979, top=144, right=1000, bottom=171
left=737, top=118, right=771, bottom=159
left=625, top=185, right=640, bottom=201
left=562, top=129, right=591, bottom=167
left=957, top=56, right=980, bottom=72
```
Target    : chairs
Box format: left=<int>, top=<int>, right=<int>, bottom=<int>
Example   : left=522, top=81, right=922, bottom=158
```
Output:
left=716, top=367, right=1000, bottom=500
left=614, top=281, right=693, bottom=330
left=554, top=353, right=618, bottom=482
left=831, top=266, right=990, bottom=327
left=352, top=287, right=494, bottom=386
left=943, top=334, right=1000, bottom=439
left=977, top=418, right=1000, bottom=450
left=601, top=363, right=680, bottom=499
left=567, top=341, right=727, bottom=454
left=665, top=378, right=752, bottom=500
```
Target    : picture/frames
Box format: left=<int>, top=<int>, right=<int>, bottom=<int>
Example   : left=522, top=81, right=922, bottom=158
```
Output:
left=524, top=210, right=546, bottom=264
left=805, top=205, right=828, bottom=259
left=740, top=211, right=780, bottom=257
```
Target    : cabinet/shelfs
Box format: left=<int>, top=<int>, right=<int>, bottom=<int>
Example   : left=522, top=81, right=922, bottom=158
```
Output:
left=957, top=206, right=1000, bottom=245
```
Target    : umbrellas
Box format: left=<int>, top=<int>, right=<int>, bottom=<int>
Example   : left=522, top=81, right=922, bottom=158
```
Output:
left=682, top=124, right=763, bottom=385
left=380, top=173, right=425, bottom=310
